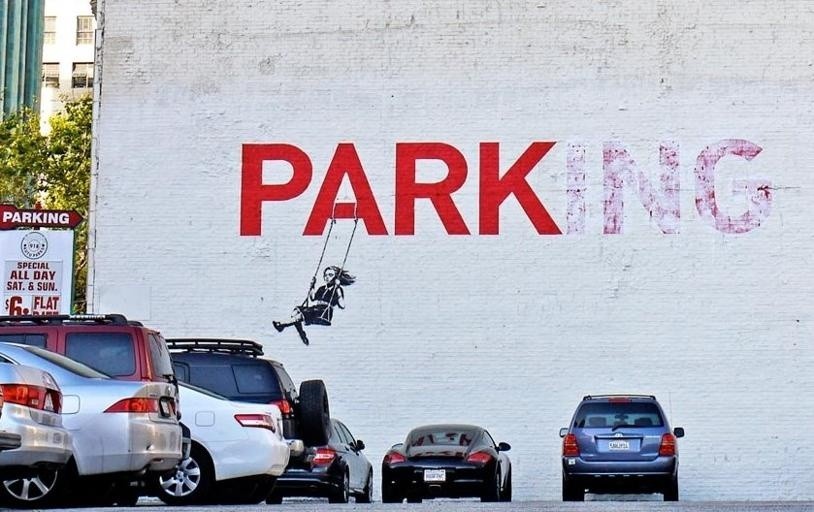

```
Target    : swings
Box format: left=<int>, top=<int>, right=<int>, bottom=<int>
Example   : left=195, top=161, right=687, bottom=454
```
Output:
left=301, top=219, right=358, bottom=325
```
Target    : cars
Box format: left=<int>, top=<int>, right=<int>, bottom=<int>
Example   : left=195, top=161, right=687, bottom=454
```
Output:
left=266, top=419, right=372, bottom=504
left=381, top=423, right=511, bottom=503
left=0, top=340, right=182, bottom=509
left=0, top=361, right=73, bottom=481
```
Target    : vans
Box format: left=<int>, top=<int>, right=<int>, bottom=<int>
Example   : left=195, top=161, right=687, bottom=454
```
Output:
left=0, top=314, right=180, bottom=507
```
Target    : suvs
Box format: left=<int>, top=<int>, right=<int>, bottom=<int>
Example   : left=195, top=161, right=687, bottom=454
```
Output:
left=558, top=394, right=684, bottom=501
left=153, top=338, right=329, bottom=506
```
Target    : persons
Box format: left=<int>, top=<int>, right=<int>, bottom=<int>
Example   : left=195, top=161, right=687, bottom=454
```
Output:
left=271, top=265, right=357, bottom=346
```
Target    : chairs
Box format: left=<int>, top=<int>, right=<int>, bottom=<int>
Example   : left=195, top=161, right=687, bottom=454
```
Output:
left=637, top=416, right=654, bottom=427
left=589, top=417, right=606, bottom=428
